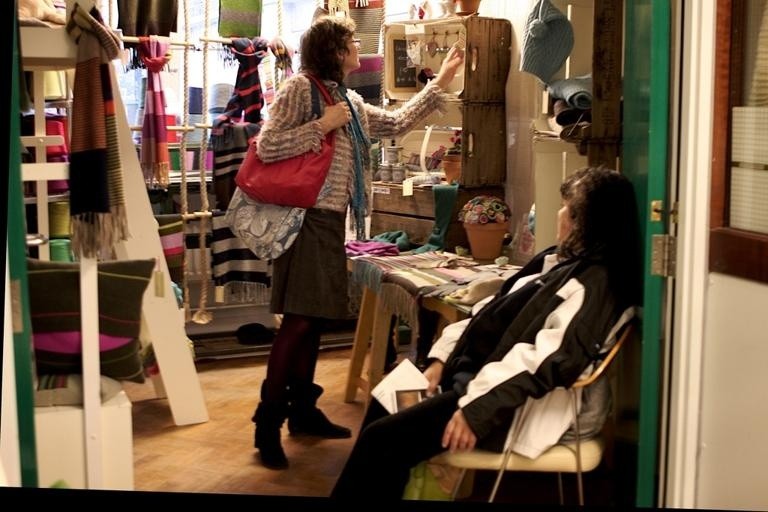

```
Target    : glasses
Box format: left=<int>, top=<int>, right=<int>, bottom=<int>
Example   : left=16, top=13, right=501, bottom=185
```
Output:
left=345, top=40, right=361, bottom=47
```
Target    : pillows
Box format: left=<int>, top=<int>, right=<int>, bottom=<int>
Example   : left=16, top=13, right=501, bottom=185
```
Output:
left=25, top=256, right=157, bottom=384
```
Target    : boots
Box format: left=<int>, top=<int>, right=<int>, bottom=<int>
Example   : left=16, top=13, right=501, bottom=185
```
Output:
left=288, top=383, right=351, bottom=438
left=252, top=380, right=292, bottom=469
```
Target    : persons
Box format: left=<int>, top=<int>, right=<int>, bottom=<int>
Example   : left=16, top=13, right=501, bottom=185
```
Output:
left=223, top=19, right=464, bottom=470
left=329, top=167, right=644, bottom=500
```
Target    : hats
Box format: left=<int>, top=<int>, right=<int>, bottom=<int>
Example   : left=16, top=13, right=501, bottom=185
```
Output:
left=518, top=0, right=574, bottom=82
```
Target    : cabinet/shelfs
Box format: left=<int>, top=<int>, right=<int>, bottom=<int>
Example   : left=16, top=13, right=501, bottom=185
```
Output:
left=369, top=17, right=512, bottom=375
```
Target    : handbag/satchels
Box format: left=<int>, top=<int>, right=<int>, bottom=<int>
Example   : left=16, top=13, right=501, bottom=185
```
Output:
left=235, top=128, right=336, bottom=208
left=224, top=186, right=307, bottom=262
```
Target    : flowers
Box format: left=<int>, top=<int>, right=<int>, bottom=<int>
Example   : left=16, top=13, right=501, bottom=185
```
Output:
left=458, top=196, right=512, bottom=224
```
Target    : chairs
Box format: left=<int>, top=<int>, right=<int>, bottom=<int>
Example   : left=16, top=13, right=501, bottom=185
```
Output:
left=429, top=322, right=630, bottom=506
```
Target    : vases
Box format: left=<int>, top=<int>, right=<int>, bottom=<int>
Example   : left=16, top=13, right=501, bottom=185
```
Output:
left=463, top=224, right=508, bottom=260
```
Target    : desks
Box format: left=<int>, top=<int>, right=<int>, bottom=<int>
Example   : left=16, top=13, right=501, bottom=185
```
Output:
left=344, top=250, right=524, bottom=417
